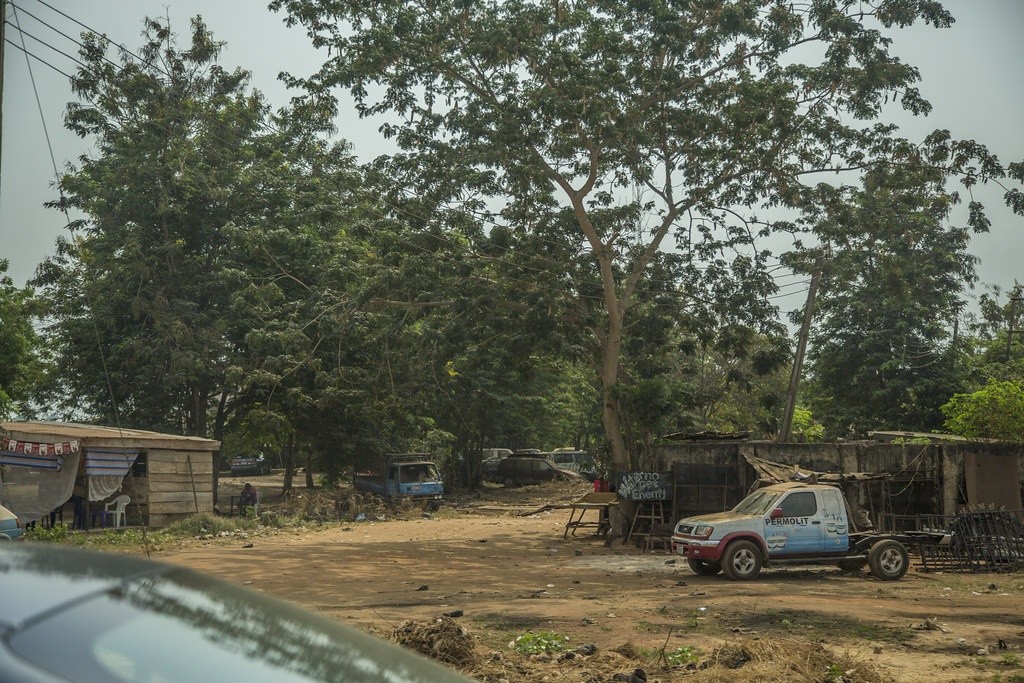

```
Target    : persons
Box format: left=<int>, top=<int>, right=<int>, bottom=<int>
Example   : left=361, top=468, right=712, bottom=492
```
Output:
left=26, top=520, right=35, bottom=530
left=237, top=483, right=256, bottom=516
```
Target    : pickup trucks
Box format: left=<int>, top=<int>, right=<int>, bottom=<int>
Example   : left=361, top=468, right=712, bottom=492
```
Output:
left=670, top=480, right=951, bottom=581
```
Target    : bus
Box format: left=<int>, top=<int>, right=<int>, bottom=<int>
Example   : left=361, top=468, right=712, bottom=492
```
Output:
left=513, top=448, right=592, bottom=473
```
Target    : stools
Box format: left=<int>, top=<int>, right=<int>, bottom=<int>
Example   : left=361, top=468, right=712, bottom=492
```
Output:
left=92, top=509, right=111, bottom=528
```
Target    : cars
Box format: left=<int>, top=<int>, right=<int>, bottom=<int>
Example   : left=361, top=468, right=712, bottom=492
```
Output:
left=495, top=456, right=583, bottom=488
left=481, top=456, right=506, bottom=472
left=229, top=453, right=272, bottom=476
left=1, top=540, right=484, bottom=683
left=0, top=505, right=26, bottom=543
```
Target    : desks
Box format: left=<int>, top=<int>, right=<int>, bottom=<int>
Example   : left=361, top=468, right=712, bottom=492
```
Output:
left=231, top=495, right=253, bottom=516
left=563, top=491, right=618, bottom=536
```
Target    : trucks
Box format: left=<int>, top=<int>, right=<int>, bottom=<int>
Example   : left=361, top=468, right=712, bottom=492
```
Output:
left=353, top=453, right=446, bottom=511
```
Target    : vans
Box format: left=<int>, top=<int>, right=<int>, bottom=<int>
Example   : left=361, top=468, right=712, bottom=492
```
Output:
left=480, top=449, right=515, bottom=462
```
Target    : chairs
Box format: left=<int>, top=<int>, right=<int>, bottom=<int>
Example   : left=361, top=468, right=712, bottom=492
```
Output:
left=72, top=496, right=95, bottom=530
left=242, top=491, right=262, bottom=517
left=394, top=470, right=408, bottom=484
left=415, top=467, right=428, bottom=482
left=101, top=494, right=131, bottom=528
